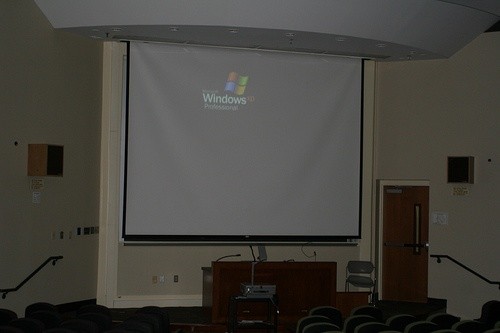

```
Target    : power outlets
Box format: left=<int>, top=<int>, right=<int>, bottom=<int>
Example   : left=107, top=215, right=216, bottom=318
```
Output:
left=173, top=275, right=179, bottom=283
left=151, top=273, right=160, bottom=284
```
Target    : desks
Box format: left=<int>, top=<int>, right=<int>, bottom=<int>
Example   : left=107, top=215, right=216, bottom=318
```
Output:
left=228, top=293, right=280, bottom=333
left=211, top=261, right=337, bottom=323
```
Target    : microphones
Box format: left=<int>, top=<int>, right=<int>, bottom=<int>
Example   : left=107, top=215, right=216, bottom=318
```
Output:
left=216, top=254, right=241, bottom=261
left=250, top=245, right=256, bottom=261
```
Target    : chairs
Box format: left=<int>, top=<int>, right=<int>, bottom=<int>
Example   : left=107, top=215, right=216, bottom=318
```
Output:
left=344, top=261, right=376, bottom=305
left=0, top=301, right=184, bottom=333
left=296, top=300, right=500, bottom=333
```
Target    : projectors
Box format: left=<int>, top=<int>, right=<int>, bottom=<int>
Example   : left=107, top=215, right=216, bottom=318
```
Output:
left=240, top=282, right=277, bottom=299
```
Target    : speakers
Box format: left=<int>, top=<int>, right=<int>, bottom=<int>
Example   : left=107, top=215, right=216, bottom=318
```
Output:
left=447, top=155, right=473, bottom=184
left=28, top=144, right=64, bottom=177
